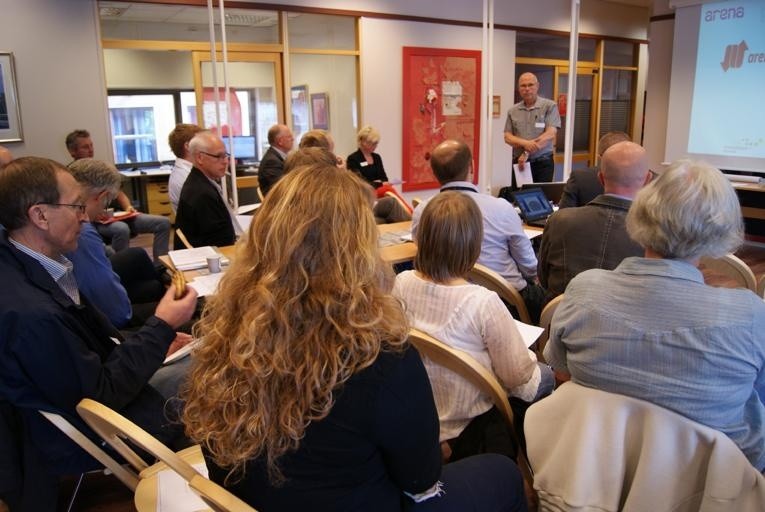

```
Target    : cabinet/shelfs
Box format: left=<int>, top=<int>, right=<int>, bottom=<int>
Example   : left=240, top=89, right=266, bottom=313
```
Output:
left=146, top=183, right=175, bottom=224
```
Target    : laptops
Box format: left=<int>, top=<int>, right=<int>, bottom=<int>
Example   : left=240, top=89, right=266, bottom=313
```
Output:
left=510, top=187, right=554, bottom=228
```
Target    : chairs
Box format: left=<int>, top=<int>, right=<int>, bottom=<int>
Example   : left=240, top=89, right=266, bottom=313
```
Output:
left=538, top=294, right=565, bottom=355
left=175, top=228, right=193, bottom=248
left=407, top=329, right=533, bottom=490
left=257, top=185, right=265, bottom=202
left=412, top=198, right=421, bottom=208
left=469, top=263, right=536, bottom=357
left=38, top=396, right=260, bottom=512
left=701, top=253, right=765, bottom=298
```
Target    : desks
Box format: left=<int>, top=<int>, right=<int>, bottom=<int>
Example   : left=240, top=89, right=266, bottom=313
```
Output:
left=119, top=161, right=259, bottom=214
left=158, top=219, right=546, bottom=299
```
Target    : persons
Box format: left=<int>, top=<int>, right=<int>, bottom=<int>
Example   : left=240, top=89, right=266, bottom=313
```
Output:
left=410, top=138, right=544, bottom=326
left=504, top=72, right=562, bottom=187
left=544, top=159, right=764, bottom=477
left=559, top=131, right=631, bottom=209
left=392, top=190, right=556, bottom=463
left=183, top=163, right=530, bottom=512
left=2, top=129, right=205, bottom=334
left=168, top=125, right=244, bottom=251
left=2, top=156, right=198, bottom=473
left=538, top=140, right=653, bottom=309
left=258, top=125, right=414, bottom=223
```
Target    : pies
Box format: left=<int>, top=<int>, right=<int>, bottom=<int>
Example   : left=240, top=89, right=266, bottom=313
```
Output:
left=173, top=269, right=185, bottom=298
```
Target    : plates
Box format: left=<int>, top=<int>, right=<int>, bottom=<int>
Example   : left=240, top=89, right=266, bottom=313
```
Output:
left=111, top=211, right=129, bottom=217
left=162, top=335, right=208, bottom=365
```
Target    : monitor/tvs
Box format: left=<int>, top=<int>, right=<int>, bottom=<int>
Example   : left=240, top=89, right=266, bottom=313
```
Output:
left=222, top=136, right=258, bottom=169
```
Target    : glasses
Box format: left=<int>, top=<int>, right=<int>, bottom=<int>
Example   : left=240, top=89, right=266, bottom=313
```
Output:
left=200, top=151, right=231, bottom=160
left=39, top=202, right=85, bottom=215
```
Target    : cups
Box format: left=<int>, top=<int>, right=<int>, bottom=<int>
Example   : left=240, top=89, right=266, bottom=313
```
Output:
left=104, top=207, right=113, bottom=218
left=205, top=254, right=223, bottom=273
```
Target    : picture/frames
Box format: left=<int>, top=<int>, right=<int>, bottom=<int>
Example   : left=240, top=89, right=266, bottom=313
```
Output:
left=0, top=51, right=24, bottom=145
left=291, top=84, right=310, bottom=137
left=310, top=93, right=329, bottom=131
